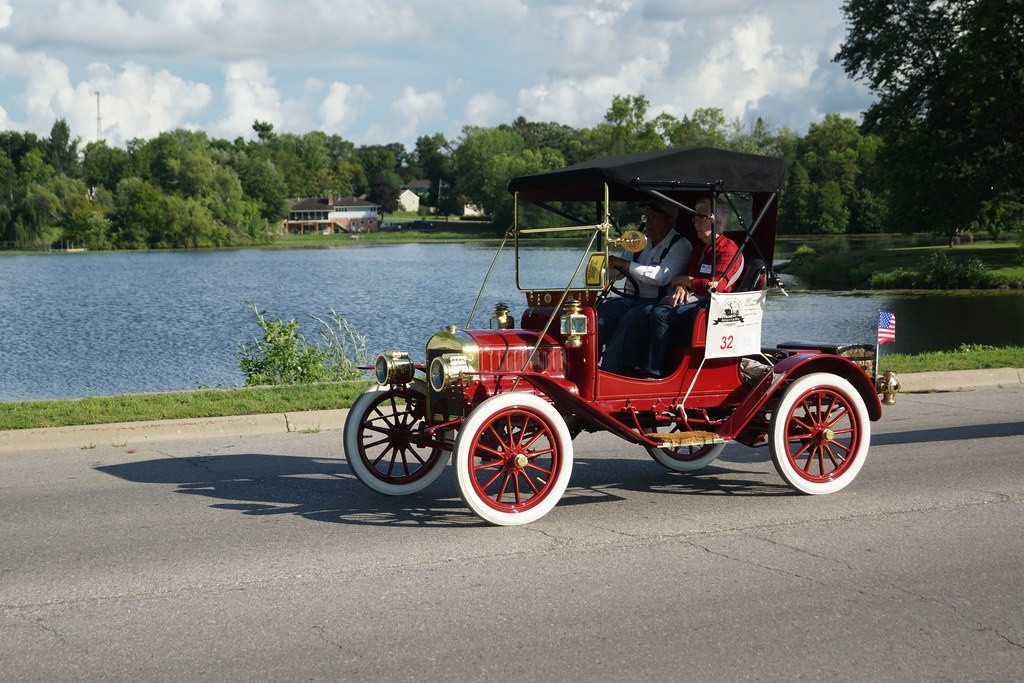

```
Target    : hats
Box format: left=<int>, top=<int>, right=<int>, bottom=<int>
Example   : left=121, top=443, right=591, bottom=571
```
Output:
left=639, top=198, right=678, bottom=219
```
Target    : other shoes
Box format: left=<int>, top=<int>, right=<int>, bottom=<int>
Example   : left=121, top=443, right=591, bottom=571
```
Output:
left=641, top=369, right=663, bottom=378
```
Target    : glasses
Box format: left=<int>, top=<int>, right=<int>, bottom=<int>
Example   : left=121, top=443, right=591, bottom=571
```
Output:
left=691, top=215, right=708, bottom=222
left=642, top=215, right=668, bottom=222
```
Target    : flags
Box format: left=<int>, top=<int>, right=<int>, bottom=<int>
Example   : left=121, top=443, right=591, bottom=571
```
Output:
left=878, top=311, right=895, bottom=344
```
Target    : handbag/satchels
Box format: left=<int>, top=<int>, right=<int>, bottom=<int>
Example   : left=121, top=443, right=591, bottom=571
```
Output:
left=659, top=289, right=705, bottom=307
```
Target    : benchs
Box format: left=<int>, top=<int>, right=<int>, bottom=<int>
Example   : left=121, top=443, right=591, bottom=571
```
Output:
left=666, top=257, right=766, bottom=349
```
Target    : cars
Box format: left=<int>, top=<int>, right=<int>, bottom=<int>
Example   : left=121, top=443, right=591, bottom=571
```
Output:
left=343, top=146, right=901, bottom=526
left=380, top=220, right=434, bottom=231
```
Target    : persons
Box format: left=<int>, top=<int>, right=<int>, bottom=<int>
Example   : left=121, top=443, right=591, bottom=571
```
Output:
left=598, top=198, right=744, bottom=378
